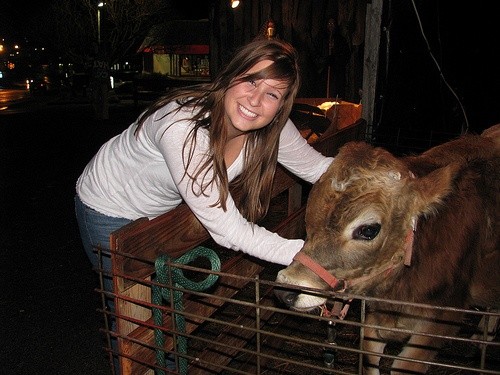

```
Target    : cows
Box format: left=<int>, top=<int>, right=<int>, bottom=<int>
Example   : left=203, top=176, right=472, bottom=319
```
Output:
left=275, top=134, right=500, bottom=375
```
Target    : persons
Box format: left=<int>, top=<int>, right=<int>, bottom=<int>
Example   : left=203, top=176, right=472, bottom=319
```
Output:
left=71, top=38, right=418, bottom=375
